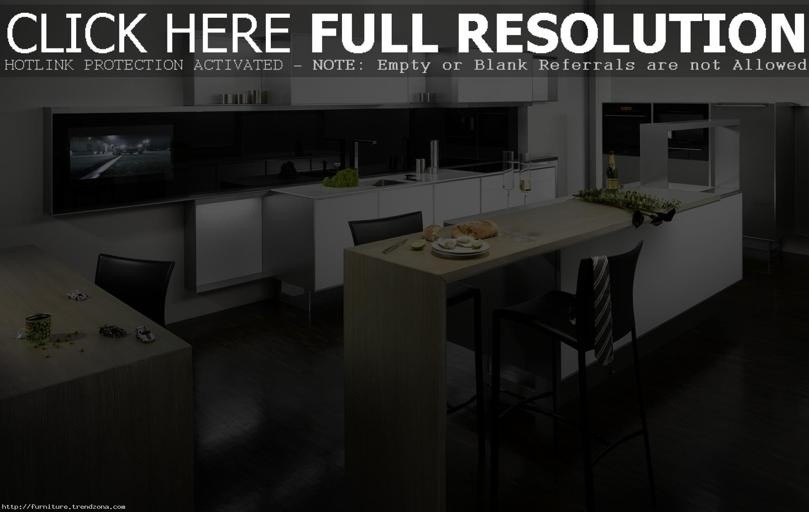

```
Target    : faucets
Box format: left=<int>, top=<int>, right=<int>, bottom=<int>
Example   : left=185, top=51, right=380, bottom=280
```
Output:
left=353, top=136, right=377, bottom=179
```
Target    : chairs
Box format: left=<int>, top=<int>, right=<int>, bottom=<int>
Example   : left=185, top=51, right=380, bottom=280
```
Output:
left=94, top=253, right=178, bottom=328
left=347, top=210, right=488, bottom=501
left=490, top=239, right=658, bottom=511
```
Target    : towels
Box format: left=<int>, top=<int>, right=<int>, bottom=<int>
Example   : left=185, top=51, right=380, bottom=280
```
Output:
left=588, top=254, right=618, bottom=368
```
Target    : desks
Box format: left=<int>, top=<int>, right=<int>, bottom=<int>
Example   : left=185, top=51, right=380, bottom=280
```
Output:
left=339, top=188, right=723, bottom=511
left=0, top=241, right=197, bottom=510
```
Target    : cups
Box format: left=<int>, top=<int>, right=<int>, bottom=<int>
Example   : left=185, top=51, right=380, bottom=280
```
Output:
left=416, top=158, right=434, bottom=182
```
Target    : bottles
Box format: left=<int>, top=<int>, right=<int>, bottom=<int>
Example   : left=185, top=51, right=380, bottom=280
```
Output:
left=607, top=150, right=619, bottom=193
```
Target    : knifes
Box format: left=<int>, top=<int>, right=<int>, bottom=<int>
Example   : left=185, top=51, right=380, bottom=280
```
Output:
left=382, top=239, right=407, bottom=255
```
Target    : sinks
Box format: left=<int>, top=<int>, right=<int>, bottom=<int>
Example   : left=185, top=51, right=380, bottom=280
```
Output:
left=372, top=177, right=409, bottom=187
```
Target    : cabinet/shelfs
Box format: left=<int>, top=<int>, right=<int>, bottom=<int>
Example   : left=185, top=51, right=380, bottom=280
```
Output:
left=425, top=51, right=534, bottom=105
left=261, top=186, right=434, bottom=294
left=180, top=31, right=263, bottom=107
left=268, top=33, right=410, bottom=103
left=434, top=178, right=481, bottom=228
left=480, top=167, right=557, bottom=214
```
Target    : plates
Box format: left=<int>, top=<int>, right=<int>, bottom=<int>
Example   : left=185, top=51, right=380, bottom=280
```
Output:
left=431, top=236, right=490, bottom=258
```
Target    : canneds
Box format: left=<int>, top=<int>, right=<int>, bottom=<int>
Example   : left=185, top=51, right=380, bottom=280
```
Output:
left=24, top=314, right=51, bottom=343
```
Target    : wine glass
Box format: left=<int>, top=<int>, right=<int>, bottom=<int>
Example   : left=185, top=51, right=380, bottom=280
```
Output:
left=502, top=151, right=514, bottom=208
left=520, top=152, right=532, bottom=207
